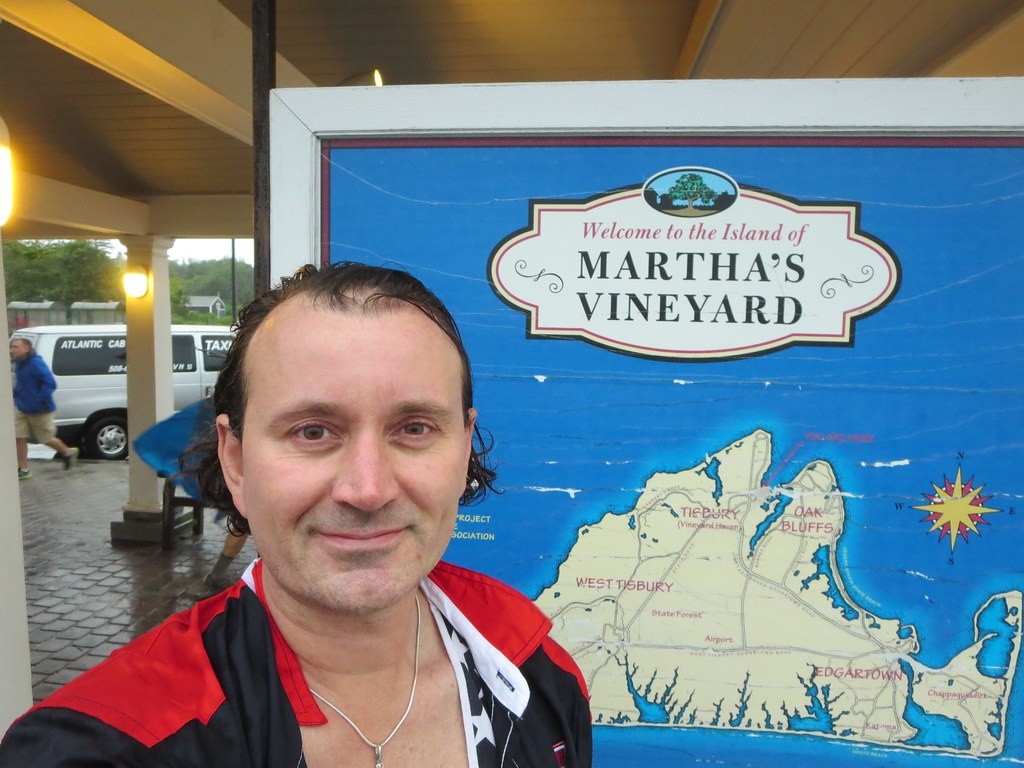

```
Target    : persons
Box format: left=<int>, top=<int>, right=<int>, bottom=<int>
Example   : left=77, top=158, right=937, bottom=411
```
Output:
left=0, top=258, right=594, bottom=768
left=9, top=338, right=80, bottom=480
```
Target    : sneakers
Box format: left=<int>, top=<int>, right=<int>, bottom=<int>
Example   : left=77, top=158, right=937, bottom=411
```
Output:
left=63, top=447, right=79, bottom=471
left=18, top=467, right=32, bottom=481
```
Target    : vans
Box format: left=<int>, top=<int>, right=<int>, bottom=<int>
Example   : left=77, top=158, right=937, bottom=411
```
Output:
left=10, top=323, right=240, bottom=463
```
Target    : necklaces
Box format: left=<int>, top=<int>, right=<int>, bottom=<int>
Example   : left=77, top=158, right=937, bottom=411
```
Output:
left=303, top=592, right=422, bottom=768
